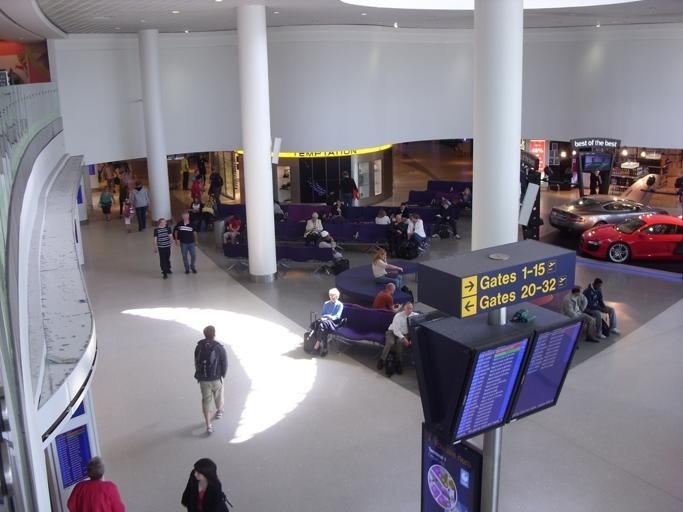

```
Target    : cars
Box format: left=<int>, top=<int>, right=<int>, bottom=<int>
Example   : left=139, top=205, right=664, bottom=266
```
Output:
left=550, top=194, right=669, bottom=236
left=579, top=213, right=683, bottom=265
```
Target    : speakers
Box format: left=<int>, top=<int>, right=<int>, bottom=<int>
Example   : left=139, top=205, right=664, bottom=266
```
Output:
left=518, top=183, right=539, bottom=226
left=271, top=136, right=281, bottom=165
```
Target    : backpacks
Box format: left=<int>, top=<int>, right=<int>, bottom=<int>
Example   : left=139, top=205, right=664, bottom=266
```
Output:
left=194, top=340, right=221, bottom=382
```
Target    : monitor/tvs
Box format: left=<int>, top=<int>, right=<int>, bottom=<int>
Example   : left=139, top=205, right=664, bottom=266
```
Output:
left=584, top=153, right=612, bottom=172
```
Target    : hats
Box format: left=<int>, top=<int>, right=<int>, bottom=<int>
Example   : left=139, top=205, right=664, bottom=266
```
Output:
left=320, top=230, right=329, bottom=238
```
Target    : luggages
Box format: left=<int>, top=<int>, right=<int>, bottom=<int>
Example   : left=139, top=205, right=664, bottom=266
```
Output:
left=304, top=331, right=316, bottom=353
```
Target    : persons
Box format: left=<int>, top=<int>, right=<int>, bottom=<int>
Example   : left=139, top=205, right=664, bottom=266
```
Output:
left=562, top=286, right=598, bottom=342
left=591, top=169, right=602, bottom=195
left=274, top=171, right=471, bottom=373
left=99, top=155, right=241, bottom=279
left=195, top=326, right=227, bottom=435
left=582, top=279, right=620, bottom=340
left=181, top=460, right=229, bottom=512
left=67, top=456, right=125, bottom=512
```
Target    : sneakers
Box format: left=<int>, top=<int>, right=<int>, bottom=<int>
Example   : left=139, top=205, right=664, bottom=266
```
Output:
left=207, top=411, right=223, bottom=433
left=376, top=359, right=403, bottom=375
left=186, top=266, right=197, bottom=274
left=162, top=270, right=171, bottom=279
left=585, top=327, right=621, bottom=343
